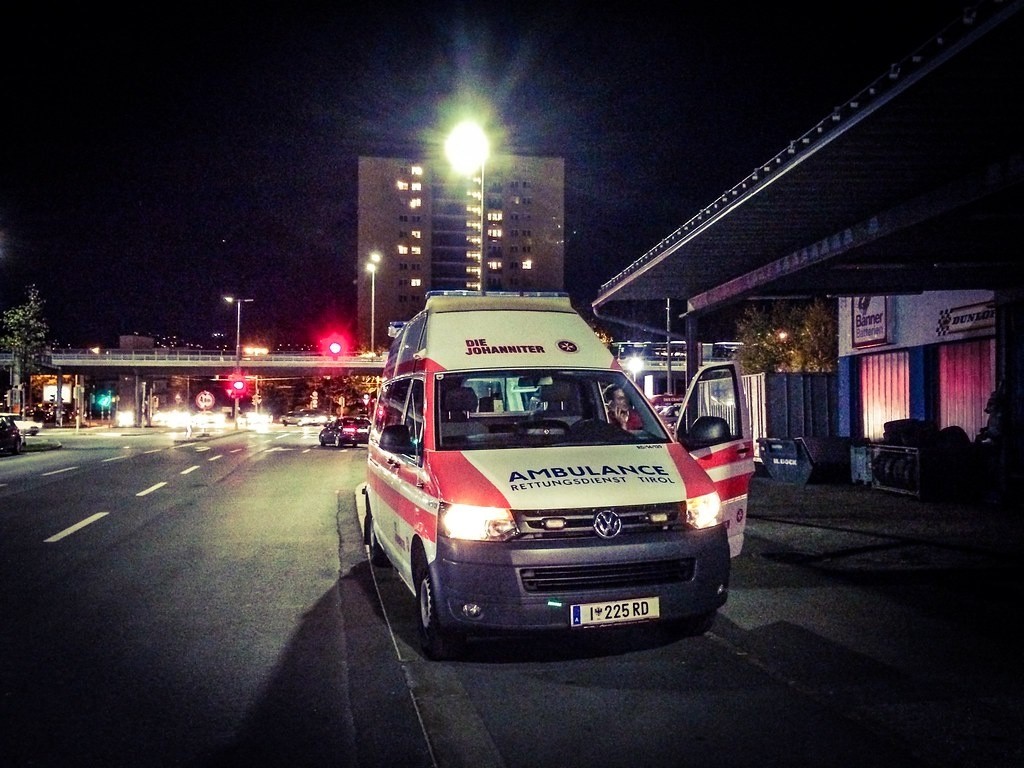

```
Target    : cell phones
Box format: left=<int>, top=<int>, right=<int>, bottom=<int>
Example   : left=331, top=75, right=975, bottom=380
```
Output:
left=609, top=400, right=619, bottom=413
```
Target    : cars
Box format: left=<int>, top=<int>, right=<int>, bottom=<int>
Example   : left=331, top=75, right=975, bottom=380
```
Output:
left=659, top=407, right=681, bottom=422
left=0, top=417, right=21, bottom=455
left=280, top=409, right=338, bottom=426
left=319, top=416, right=372, bottom=447
left=0, top=413, right=41, bottom=436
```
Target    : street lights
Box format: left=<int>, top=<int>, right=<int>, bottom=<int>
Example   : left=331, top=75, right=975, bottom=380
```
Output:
left=223, top=295, right=254, bottom=360
left=243, top=346, right=268, bottom=413
left=444, top=122, right=490, bottom=288
left=365, top=252, right=383, bottom=353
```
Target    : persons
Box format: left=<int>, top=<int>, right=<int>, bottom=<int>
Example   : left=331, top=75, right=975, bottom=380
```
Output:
left=601, top=382, right=644, bottom=431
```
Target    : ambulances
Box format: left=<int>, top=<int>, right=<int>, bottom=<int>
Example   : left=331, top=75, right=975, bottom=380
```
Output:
left=363, top=290, right=756, bottom=663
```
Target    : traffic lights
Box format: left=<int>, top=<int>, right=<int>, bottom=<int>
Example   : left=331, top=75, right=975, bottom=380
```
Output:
left=50, top=394, right=54, bottom=406
left=4, top=390, right=10, bottom=407
left=101, top=396, right=109, bottom=405
left=331, top=341, right=341, bottom=359
left=233, top=379, right=245, bottom=395
